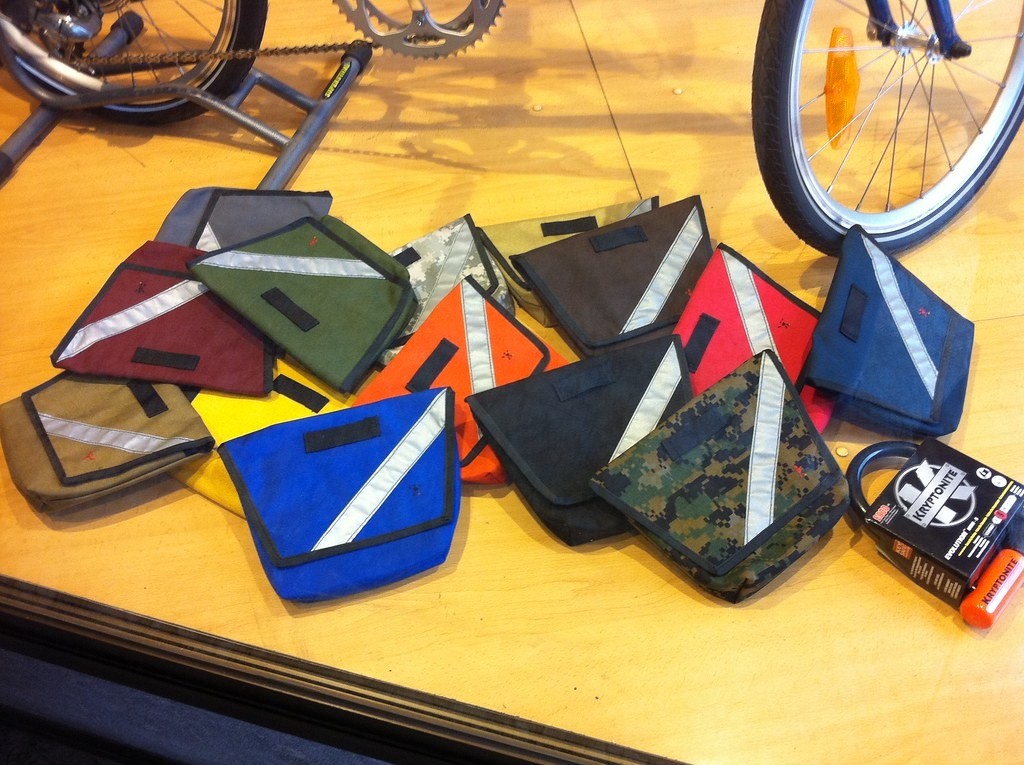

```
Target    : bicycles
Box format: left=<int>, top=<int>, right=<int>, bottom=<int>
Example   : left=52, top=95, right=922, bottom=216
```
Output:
left=0, top=0, right=1024, bottom=255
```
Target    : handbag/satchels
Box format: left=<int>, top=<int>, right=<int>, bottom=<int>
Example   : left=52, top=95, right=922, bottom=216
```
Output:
left=0, top=186, right=975, bottom=606
